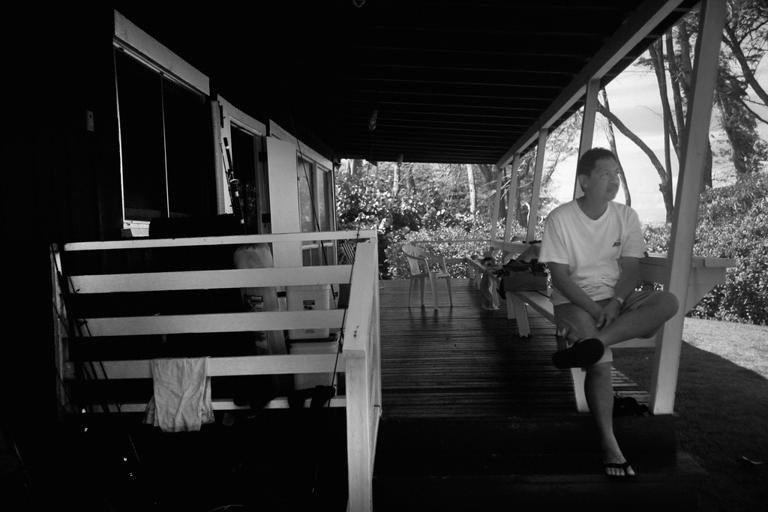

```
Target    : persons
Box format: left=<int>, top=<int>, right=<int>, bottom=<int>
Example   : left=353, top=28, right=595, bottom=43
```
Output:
left=538, top=147, right=680, bottom=479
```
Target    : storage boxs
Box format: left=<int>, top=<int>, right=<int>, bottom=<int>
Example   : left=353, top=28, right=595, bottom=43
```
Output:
left=285, top=332, right=340, bottom=397
left=284, top=284, right=331, bottom=340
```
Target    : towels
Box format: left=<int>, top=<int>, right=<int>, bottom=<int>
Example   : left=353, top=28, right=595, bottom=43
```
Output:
left=141, top=354, right=217, bottom=434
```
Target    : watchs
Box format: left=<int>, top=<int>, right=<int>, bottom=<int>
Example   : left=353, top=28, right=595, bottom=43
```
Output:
left=612, top=295, right=625, bottom=306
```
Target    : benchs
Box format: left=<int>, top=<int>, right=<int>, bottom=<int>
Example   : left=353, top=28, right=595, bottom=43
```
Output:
left=464, top=239, right=736, bottom=415
left=47, top=230, right=382, bottom=511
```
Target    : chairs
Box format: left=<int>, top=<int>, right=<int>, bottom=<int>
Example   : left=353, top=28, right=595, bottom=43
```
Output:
left=402, top=245, right=453, bottom=311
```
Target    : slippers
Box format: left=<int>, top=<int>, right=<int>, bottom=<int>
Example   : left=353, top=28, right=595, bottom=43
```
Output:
left=551, top=335, right=604, bottom=372
left=594, top=442, right=638, bottom=484
